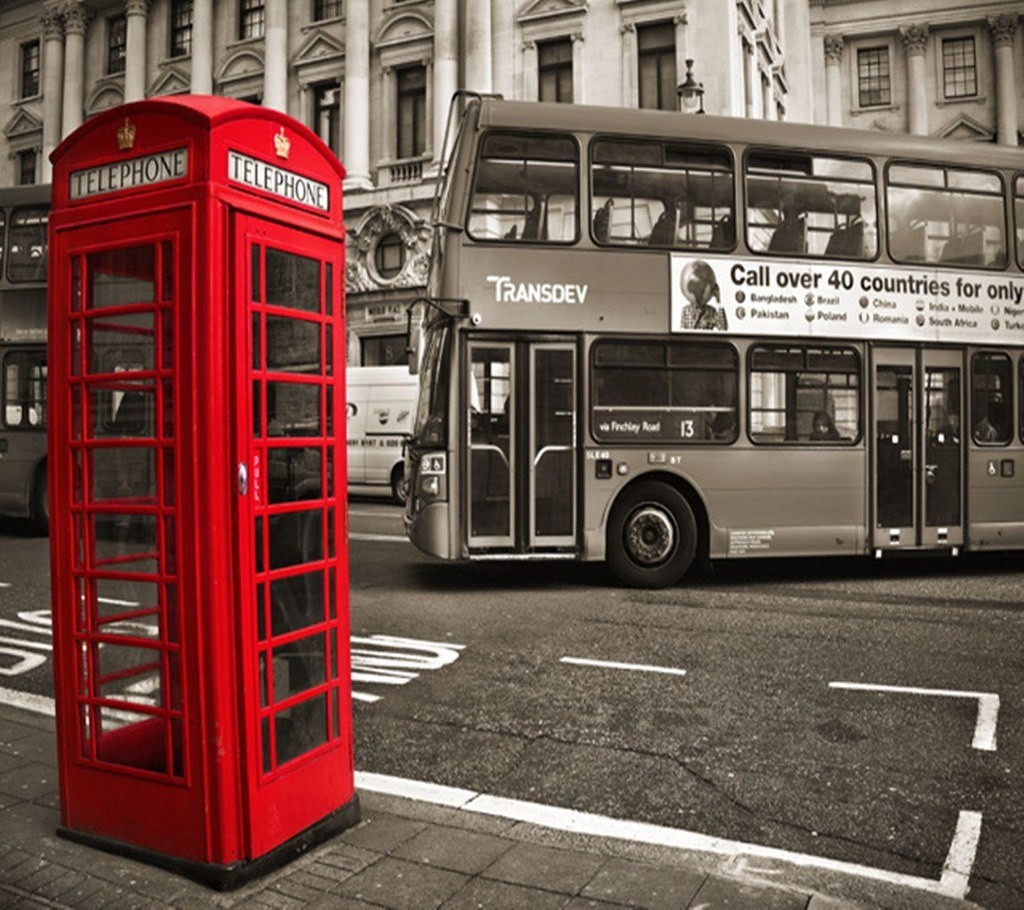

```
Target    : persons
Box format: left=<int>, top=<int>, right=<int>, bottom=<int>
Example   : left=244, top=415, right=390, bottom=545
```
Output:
left=714, top=399, right=738, bottom=440
left=810, top=411, right=839, bottom=441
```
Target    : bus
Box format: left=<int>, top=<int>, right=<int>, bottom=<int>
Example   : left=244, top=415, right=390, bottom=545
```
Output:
left=0, top=184, right=164, bottom=540
left=395, top=92, right=1024, bottom=592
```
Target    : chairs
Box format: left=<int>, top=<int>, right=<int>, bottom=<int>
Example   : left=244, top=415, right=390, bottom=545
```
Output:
left=522, top=201, right=1024, bottom=445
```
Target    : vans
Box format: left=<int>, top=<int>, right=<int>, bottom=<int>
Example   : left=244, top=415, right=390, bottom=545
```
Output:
left=342, top=365, right=477, bottom=503
